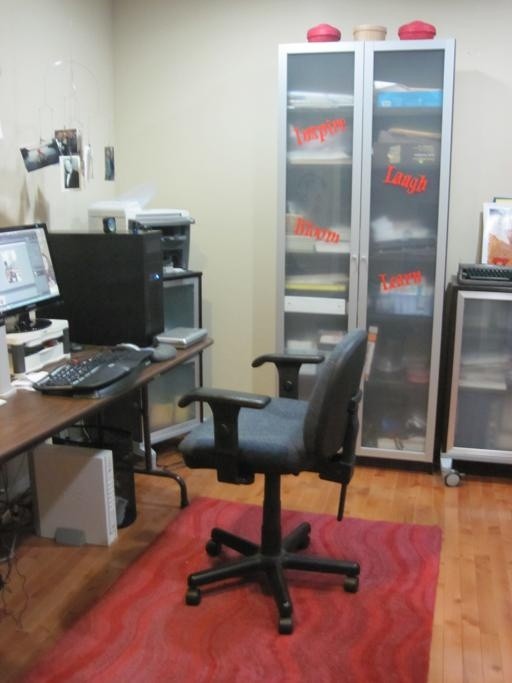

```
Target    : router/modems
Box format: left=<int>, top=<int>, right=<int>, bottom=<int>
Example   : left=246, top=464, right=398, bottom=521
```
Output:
left=153, top=326, right=207, bottom=350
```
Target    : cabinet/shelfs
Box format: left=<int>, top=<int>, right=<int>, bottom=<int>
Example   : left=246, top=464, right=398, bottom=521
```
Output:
left=439, top=276, right=512, bottom=487
left=88, top=268, right=206, bottom=472
left=278, top=35, right=456, bottom=463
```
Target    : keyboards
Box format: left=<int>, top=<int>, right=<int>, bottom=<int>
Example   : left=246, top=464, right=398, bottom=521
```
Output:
left=32, top=349, right=153, bottom=390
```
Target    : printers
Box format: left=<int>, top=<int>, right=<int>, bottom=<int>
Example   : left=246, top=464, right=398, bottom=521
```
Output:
left=88, top=205, right=195, bottom=274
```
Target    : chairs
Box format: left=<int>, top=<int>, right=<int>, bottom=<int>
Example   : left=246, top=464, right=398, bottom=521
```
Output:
left=178, top=330, right=369, bottom=631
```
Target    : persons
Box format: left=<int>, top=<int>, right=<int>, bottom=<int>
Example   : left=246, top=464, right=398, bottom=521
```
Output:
left=105, top=149, right=114, bottom=180
left=55, top=129, right=78, bottom=157
left=62, top=159, right=79, bottom=188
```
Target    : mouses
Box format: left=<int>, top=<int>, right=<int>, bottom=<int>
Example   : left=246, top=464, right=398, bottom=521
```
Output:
left=112, top=344, right=140, bottom=351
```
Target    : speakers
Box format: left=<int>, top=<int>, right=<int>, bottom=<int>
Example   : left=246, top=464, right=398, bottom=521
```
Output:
left=35, top=230, right=164, bottom=348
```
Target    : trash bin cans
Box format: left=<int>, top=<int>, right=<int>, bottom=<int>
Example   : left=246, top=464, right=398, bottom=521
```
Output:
left=52, top=424, right=137, bottom=529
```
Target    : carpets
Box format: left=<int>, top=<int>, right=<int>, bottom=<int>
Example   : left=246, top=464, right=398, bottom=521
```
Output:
left=19, top=497, right=443, bottom=682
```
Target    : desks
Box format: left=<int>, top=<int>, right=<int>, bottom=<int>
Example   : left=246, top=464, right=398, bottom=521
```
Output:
left=1, top=339, right=234, bottom=503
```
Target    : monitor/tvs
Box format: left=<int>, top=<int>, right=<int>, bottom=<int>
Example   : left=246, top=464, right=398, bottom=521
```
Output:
left=0, top=223, right=61, bottom=333
